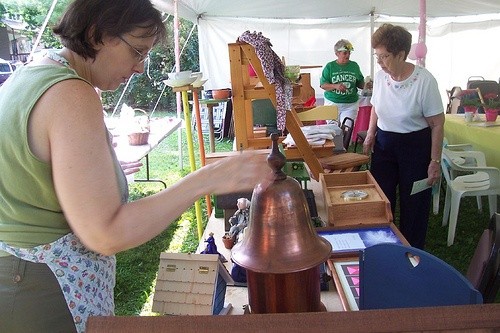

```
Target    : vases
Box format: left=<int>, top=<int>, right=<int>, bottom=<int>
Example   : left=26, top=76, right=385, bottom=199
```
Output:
left=464, top=107, right=477, bottom=122
left=484, top=108, right=499, bottom=121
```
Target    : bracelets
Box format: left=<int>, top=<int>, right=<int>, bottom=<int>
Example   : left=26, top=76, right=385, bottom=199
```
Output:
left=432, top=159, right=441, bottom=164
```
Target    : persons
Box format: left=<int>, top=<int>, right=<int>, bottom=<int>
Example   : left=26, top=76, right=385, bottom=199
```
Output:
left=0, top=0, right=275, bottom=333
left=319, top=39, right=373, bottom=150
left=363, top=23, right=445, bottom=250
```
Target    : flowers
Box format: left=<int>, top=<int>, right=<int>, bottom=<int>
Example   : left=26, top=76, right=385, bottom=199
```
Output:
left=484, top=92, right=498, bottom=109
left=459, top=89, right=478, bottom=108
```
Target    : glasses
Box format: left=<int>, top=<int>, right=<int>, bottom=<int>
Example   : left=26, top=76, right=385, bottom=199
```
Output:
left=115, top=33, right=149, bottom=66
left=372, top=53, right=391, bottom=59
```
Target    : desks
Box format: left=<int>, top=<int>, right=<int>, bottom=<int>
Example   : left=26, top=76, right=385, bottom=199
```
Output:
left=106, top=118, right=182, bottom=196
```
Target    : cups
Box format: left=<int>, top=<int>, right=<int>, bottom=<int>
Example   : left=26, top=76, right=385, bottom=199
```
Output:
left=465, top=112, right=473, bottom=123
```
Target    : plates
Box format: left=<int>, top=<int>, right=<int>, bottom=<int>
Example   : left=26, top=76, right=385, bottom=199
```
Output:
left=163, top=77, right=197, bottom=87
left=191, top=79, right=208, bottom=87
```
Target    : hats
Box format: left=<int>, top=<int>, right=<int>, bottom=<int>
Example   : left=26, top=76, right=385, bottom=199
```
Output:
left=338, top=45, right=355, bottom=51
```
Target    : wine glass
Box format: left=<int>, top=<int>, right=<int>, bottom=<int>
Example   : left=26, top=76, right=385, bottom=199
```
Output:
left=285, top=65, right=300, bottom=85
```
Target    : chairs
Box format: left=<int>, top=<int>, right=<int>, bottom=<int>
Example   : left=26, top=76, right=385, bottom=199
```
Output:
left=354, top=131, right=374, bottom=168
left=432, top=137, right=500, bottom=247
left=340, top=117, right=354, bottom=151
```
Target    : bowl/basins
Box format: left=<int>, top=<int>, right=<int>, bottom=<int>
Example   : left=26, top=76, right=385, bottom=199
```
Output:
left=168, top=71, right=191, bottom=80
left=212, top=90, right=229, bottom=100
left=192, top=72, right=202, bottom=81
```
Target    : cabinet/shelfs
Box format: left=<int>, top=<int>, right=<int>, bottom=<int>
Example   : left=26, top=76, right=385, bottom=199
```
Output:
left=227, top=43, right=338, bottom=158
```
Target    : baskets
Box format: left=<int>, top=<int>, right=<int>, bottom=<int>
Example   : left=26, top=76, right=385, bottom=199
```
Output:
left=128, top=109, right=150, bottom=145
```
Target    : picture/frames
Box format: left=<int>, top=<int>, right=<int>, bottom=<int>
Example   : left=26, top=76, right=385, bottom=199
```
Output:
left=327, top=258, right=360, bottom=312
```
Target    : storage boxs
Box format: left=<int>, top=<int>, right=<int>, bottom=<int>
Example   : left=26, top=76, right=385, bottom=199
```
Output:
left=319, top=172, right=393, bottom=226
